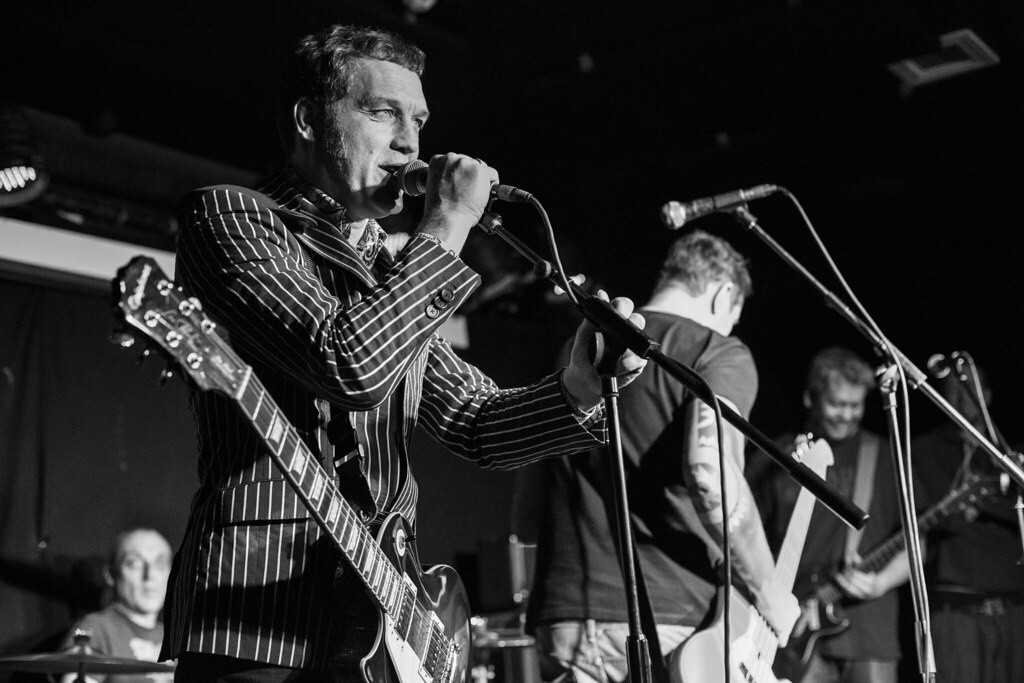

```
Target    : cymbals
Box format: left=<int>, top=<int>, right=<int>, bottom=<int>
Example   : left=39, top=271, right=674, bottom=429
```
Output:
left=1, top=642, right=176, bottom=676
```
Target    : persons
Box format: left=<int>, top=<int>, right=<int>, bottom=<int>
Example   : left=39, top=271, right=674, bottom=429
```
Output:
left=58, top=525, right=173, bottom=683
left=173, top=25, right=653, bottom=682
left=525, top=229, right=802, bottom=682
left=742, top=349, right=925, bottom=682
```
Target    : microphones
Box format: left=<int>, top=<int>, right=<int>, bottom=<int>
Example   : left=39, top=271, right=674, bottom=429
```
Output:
left=397, top=159, right=533, bottom=203
left=927, top=350, right=964, bottom=379
left=661, top=183, right=778, bottom=230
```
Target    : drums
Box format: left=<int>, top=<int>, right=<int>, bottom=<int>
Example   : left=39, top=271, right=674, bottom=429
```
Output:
left=469, top=631, right=540, bottom=683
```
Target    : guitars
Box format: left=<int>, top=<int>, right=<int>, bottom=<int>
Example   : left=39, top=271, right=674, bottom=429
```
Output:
left=770, top=471, right=1011, bottom=683
left=669, top=432, right=835, bottom=683
left=111, top=254, right=472, bottom=683
